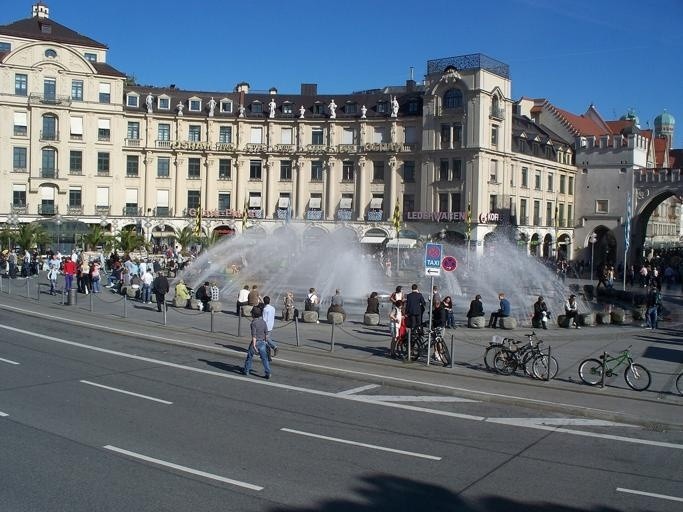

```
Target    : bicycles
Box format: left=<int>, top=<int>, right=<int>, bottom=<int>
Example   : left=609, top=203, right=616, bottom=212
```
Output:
left=483, top=331, right=548, bottom=378
left=675, top=372, right=682, bottom=396
left=393, top=320, right=450, bottom=366
left=493, top=340, right=558, bottom=381
left=578, top=345, right=652, bottom=392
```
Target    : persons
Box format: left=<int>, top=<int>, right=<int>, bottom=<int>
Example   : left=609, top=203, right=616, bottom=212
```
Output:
left=488, top=294, right=511, bottom=329
left=360, top=104, right=367, bottom=115
left=284, top=293, right=294, bottom=308
left=299, top=105, right=305, bottom=114
left=261, top=297, right=279, bottom=355
left=362, top=250, right=408, bottom=277
left=391, top=284, right=426, bottom=330
left=628, top=263, right=675, bottom=291
left=596, top=264, right=615, bottom=289
left=145, top=91, right=154, bottom=109
left=327, top=289, right=346, bottom=320
left=466, top=295, right=485, bottom=318
left=267, top=98, right=276, bottom=113
left=555, top=259, right=585, bottom=276
left=643, top=287, right=659, bottom=330
left=205, top=96, right=217, bottom=112
left=564, top=295, right=582, bottom=329
left=534, top=296, right=551, bottom=330
left=433, top=285, right=455, bottom=329
left=366, top=292, right=380, bottom=314
left=175, top=100, right=184, bottom=112
left=176, top=279, right=193, bottom=308
left=238, top=307, right=271, bottom=379
left=154, top=271, right=170, bottom=312
left=238, top=104, right=244, bottom=113
left=2, top=248, right=103, bottom=296
left=327, top=99, right=336, bottom=115
left=195, top=282, right=220, bottom=310
left=304, top=287, right=319, bottom=312
left=389, top=94, right=399, bottom=114
left=236, top=285, right=259, bottom=307
left=106, top=251, right=196, bottom=304
left=387, top=300, right=402, bottom=360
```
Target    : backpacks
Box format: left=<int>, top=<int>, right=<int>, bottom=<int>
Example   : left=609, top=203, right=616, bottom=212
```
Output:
left=305, top=295, right=313, bottom=311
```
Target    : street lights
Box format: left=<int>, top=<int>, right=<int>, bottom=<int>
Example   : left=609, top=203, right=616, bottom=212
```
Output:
left=6, top=213, right=11, bottom=251
left=357, top=223, right=363, bottom=255
left=143, top=218, right=258, bottom=262
left=439, top=228, right=445, bottom=243
left=100, top=212, right=108, bottom=251
left=111, top=219, right=118, bottom=252
left=588, top=231, right=597, bottom=285
left=12, top=213, right=19, bottom=253
left=564, top=235, right=569, bottom=257
left=54, top=213, right=62, bottom=251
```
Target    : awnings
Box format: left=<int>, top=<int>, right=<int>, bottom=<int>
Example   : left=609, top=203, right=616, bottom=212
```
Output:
left=360, top=237, right=385, bottom=244
left=386, top=238, right=417, bottom=248
